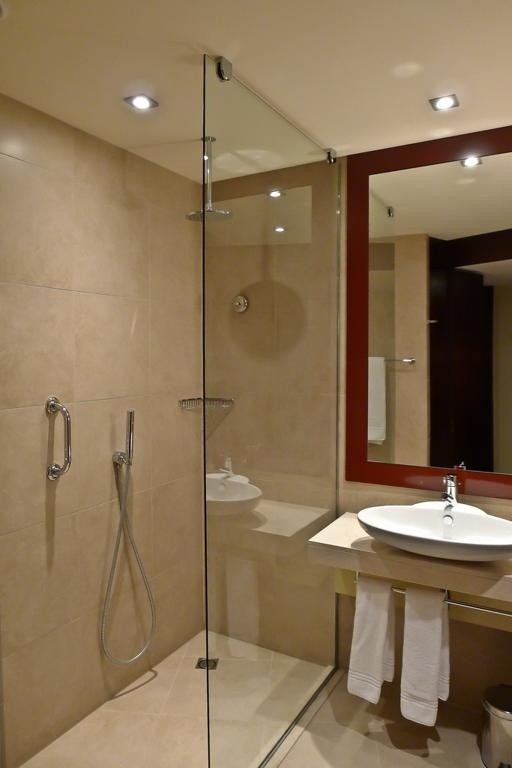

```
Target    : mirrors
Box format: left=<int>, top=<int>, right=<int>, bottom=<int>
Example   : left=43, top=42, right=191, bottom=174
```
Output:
left=347, top=125, right=512, bottom=500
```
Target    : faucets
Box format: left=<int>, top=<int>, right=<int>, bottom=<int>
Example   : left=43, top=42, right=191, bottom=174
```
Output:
left=440, top=472, right=457, bottom=505
left=455, top=460, right=467, bottom=469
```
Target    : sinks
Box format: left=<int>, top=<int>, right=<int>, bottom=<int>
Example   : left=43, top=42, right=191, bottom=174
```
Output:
left=205, top=469, right=264, bottom=516
left=359, top=475, right=512, bottom=562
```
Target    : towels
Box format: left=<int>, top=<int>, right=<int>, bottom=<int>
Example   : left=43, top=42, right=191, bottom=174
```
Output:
left=346, top=575, right=450, bottom=727
left=367, top=356, right=386, bottom=446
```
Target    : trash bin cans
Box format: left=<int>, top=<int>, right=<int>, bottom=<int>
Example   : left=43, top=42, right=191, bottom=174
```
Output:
left=480, top=684, right=512, bottom=768
